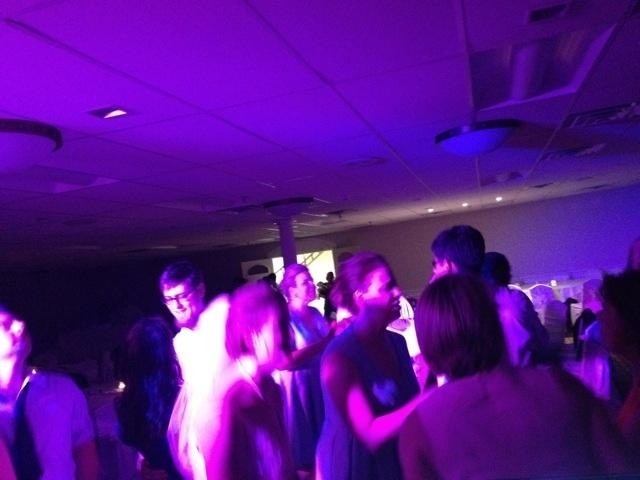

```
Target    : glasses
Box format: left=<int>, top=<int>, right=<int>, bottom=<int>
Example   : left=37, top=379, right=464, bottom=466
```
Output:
left=160, top=286, right=198, bottom=305
left=432, top=258, right=443, bottom=269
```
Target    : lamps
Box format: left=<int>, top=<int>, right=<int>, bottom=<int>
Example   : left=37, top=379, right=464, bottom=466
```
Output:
left=0, top=119, right=63, bottom=164
left=433, top=119, right=523, bottom=158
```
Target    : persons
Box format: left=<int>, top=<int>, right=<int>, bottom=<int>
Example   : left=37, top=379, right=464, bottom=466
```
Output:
left=152, top=249, right=639, bottom=480
left=427, top=224, right=525, bottom=370
left=1, top=302, right=105, bottom=480
left=110, top=313, right=185, bottom=480
